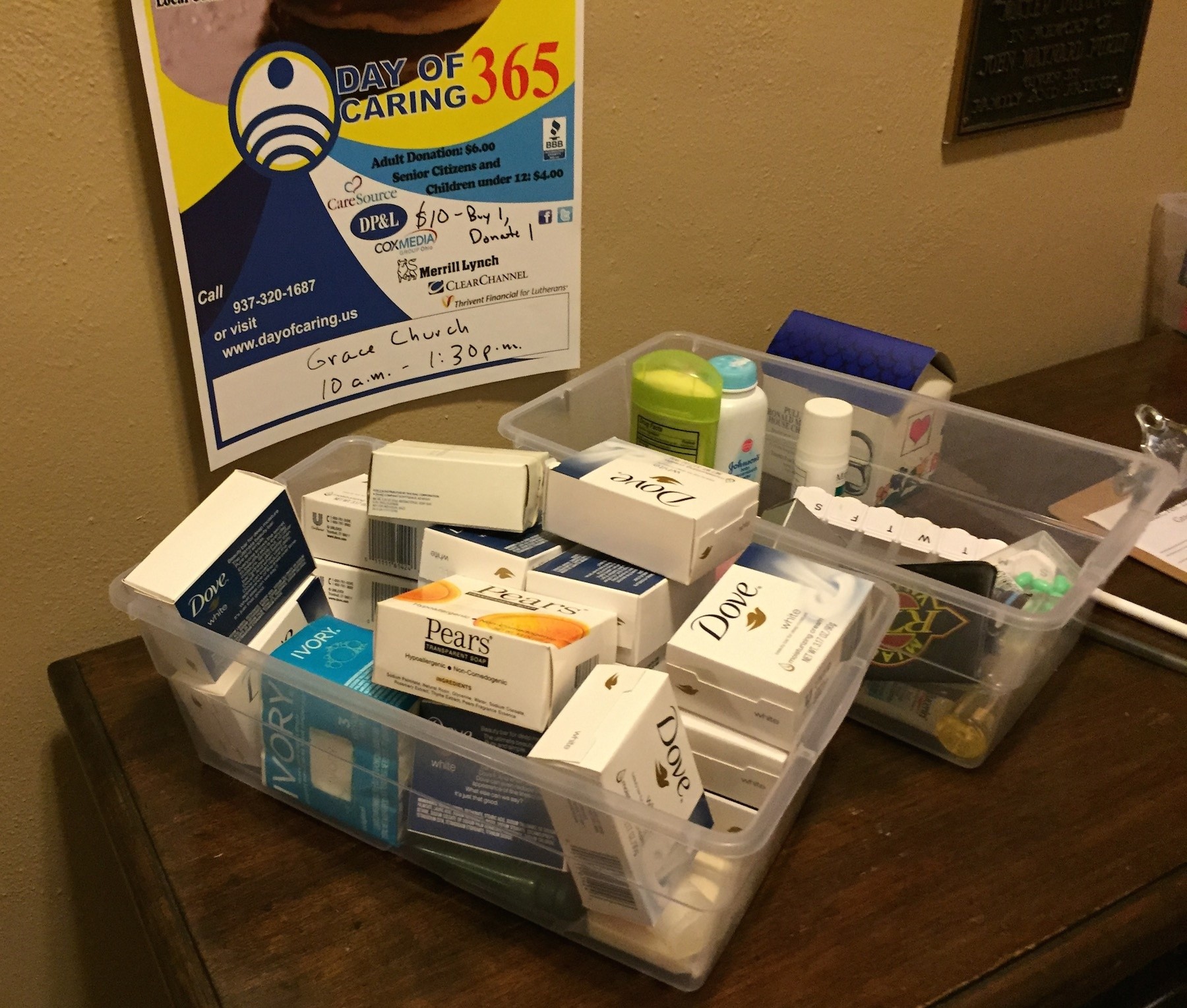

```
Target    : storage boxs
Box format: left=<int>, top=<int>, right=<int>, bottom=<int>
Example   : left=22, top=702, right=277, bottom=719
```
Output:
left=106, top=327, right=1179, bottom=992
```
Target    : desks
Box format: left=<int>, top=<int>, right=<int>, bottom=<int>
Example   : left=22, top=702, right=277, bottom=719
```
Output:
left=47, top=309, right=1187, bottom=1008
